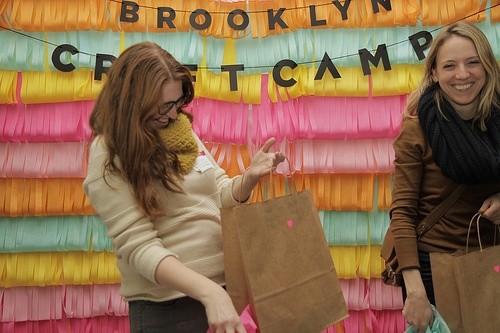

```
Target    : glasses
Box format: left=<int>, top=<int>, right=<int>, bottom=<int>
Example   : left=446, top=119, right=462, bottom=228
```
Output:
left=157, top=95, right=188, bottom=115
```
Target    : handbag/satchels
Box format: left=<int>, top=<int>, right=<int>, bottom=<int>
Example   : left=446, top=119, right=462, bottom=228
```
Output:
left=430, top=212, right=500, bottom=333
left=218, top=155, right=350, bottom=333
left=379, top=227, right=404, bottom=287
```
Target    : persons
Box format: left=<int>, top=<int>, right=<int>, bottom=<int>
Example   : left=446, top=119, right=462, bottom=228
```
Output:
left=82, top=42, right=287, bottom=333
left=389, top=22, right=500, bottom=333
